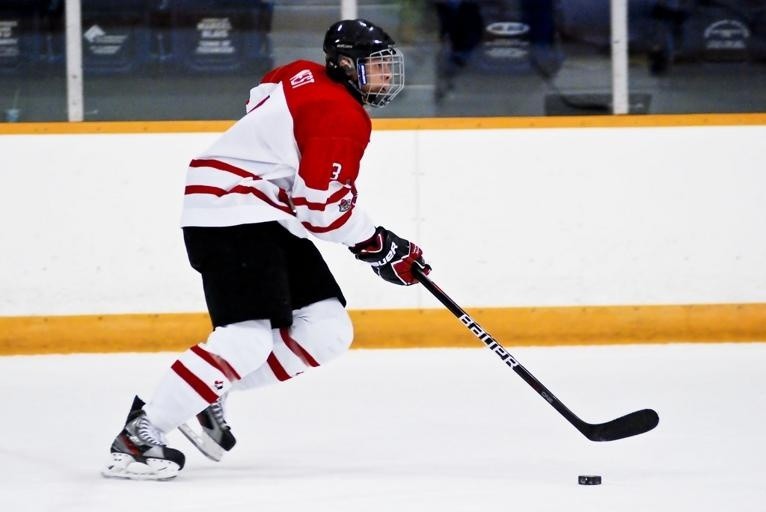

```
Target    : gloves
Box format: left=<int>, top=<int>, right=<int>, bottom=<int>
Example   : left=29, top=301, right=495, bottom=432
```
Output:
left=349, top=226, right=433, bottom=287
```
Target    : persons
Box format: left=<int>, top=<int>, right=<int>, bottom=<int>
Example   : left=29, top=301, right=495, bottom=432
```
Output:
left=106, top=18, right=433, bottom=482
left=0, top=1, right=766, bottom=127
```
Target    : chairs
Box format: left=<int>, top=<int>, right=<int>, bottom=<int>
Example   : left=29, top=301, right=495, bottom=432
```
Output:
left=0, top=1, right=277, bottom=81
left=433, top=0, right=765, bottom=81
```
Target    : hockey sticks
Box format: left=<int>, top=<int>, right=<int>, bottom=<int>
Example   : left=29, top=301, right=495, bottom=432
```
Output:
left=412, top=268, right=659, bottom=442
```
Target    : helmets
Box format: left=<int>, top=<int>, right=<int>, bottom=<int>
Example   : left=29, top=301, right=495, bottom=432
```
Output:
left=322, top=18, right=407, bottom=109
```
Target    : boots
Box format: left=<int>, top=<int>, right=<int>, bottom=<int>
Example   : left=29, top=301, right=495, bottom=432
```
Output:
left=188, top=397, right=238, bottom=451
left=109, top=393, right=186, bottom=469
